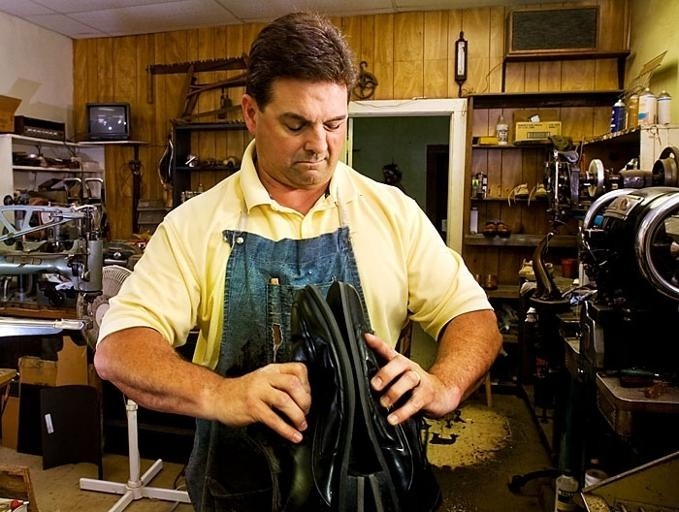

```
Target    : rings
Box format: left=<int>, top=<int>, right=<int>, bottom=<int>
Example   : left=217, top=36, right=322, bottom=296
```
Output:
left=411, top=369, right=421, bottom=382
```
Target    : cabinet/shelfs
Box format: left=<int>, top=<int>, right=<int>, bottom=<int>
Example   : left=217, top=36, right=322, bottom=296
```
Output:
left=171, top=120, right=248, bottom=208
left=580, top=123, right=678, bottom=187
left=0, top=132, right=107, bottom=245
left=464, top=89, right=628, bottom=388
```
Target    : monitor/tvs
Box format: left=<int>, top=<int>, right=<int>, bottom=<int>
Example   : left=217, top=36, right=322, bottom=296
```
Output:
left=87, top=102, right=131, bottom=140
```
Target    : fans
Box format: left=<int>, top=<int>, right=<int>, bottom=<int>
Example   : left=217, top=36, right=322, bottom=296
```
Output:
left=76, top=265, right=193, bottom=512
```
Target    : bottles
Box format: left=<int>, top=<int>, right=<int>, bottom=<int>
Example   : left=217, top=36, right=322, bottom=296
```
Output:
left=555, top=469, right=578, bottom=512
left=625, top=91, right=639, bottom=128
left=496, top=115, right=508, bottom=145
left=658, top=90, right=671, bottom=124
left=610, top=99, right=626, bottom=132
left=585, top=458, right=608, bottom=488
left=638, top=87, right=657, bottom=125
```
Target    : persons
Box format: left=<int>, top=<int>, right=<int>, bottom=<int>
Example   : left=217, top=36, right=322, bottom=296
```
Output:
left=95, top=10, right=504, bottom=510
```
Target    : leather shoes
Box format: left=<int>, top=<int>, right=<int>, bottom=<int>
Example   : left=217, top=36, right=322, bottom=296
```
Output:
left=475, top=274, right=497, bottom=290
left=483, top=223, right=509, bottom=235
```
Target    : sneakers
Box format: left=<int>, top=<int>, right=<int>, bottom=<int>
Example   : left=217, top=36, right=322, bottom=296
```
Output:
left=514, top=183, right=530, bottom=198
left=534, top=184, right=546, bottom=198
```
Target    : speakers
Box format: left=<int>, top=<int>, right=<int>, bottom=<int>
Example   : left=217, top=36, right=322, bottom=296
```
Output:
left=40, top=384, right=100, bottom=471
